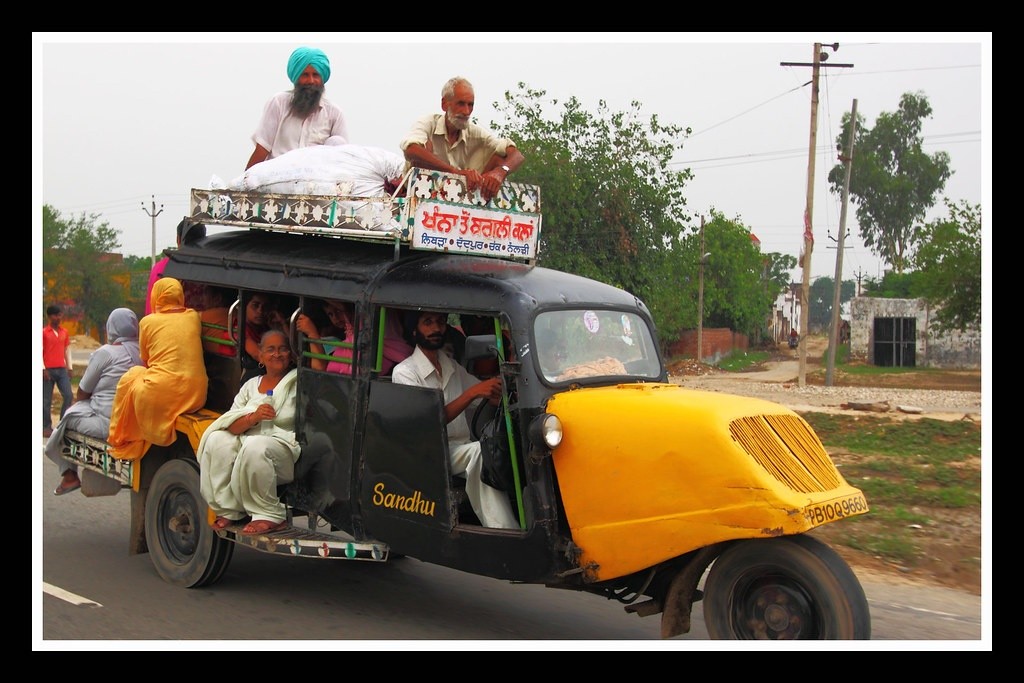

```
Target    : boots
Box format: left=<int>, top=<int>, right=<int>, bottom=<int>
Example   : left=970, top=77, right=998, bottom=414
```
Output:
left=54, top=483, right=81, bottom=495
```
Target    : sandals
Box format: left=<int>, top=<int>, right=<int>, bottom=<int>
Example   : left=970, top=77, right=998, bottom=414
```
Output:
left=211, top=516, right=232, bottom=530
left=243, top=520, right=287, bottom=535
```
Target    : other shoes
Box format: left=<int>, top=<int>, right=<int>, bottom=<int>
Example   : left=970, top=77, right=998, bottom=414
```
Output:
left=44, top=429, right=53, bottom=438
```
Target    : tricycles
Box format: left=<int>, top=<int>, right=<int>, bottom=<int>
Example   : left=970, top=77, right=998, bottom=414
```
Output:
left=52, top=159, right=875, bottom=640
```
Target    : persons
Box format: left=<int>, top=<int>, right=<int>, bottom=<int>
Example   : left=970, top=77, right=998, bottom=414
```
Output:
left=193, top=283, right=466, bottom=382
left=195, top=328, right=328, bottom=536
left=44, top=307, right=144, bottom=496
left=245, top=45, right=349, bottom=172
left=44, top=304, right=75, bottom=438
left=391, top=310, right=522, bottom=532
left=791, top=328, right=798, bottom=341
left=106, top=278, right=210, bottom=462
left=143, top=219, right=209, bottom=311
left=398, top=77, right=527, bottom=200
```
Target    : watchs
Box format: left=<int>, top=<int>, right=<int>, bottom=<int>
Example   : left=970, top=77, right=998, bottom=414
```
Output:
left=498, top=164, right=510, bottom=175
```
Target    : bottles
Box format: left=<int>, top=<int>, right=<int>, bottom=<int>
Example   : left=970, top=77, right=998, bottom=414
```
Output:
left=261, top=389, right=275, bottom=436
